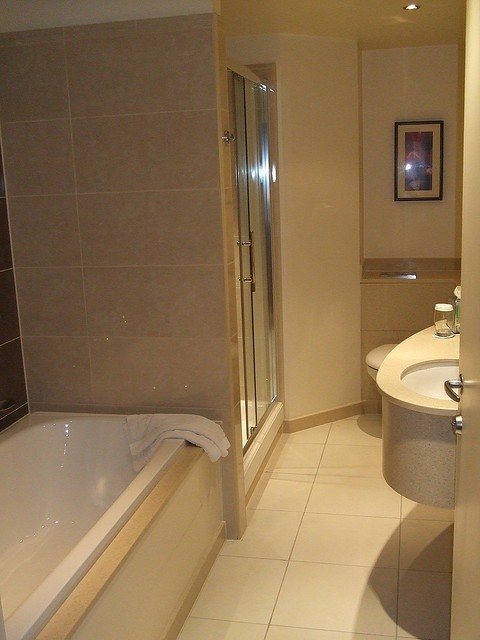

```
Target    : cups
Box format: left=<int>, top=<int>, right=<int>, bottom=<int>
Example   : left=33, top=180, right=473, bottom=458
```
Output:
left=433, top=303, right=455, bottom=337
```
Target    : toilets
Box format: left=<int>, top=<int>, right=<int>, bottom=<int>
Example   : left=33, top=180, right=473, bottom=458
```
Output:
left=365, top=344, right=398, bottom=382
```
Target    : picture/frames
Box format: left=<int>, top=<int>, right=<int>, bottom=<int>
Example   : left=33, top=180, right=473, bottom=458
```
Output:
left=393, top=120, right=444, bottom=201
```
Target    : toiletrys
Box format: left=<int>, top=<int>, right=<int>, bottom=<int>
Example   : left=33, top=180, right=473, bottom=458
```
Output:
left=452, top=285, right=461, bottom=334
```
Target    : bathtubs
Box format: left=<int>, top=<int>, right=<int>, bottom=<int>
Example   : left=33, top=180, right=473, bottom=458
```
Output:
left=0, top=411, right=225, bottom=640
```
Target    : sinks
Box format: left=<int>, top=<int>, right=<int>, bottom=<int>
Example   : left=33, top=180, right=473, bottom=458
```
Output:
left=400, top=359, right=459, bottom=400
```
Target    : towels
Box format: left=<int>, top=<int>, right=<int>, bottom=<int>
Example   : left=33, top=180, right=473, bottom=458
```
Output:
left=125, top=413, right=232, bottom=473
